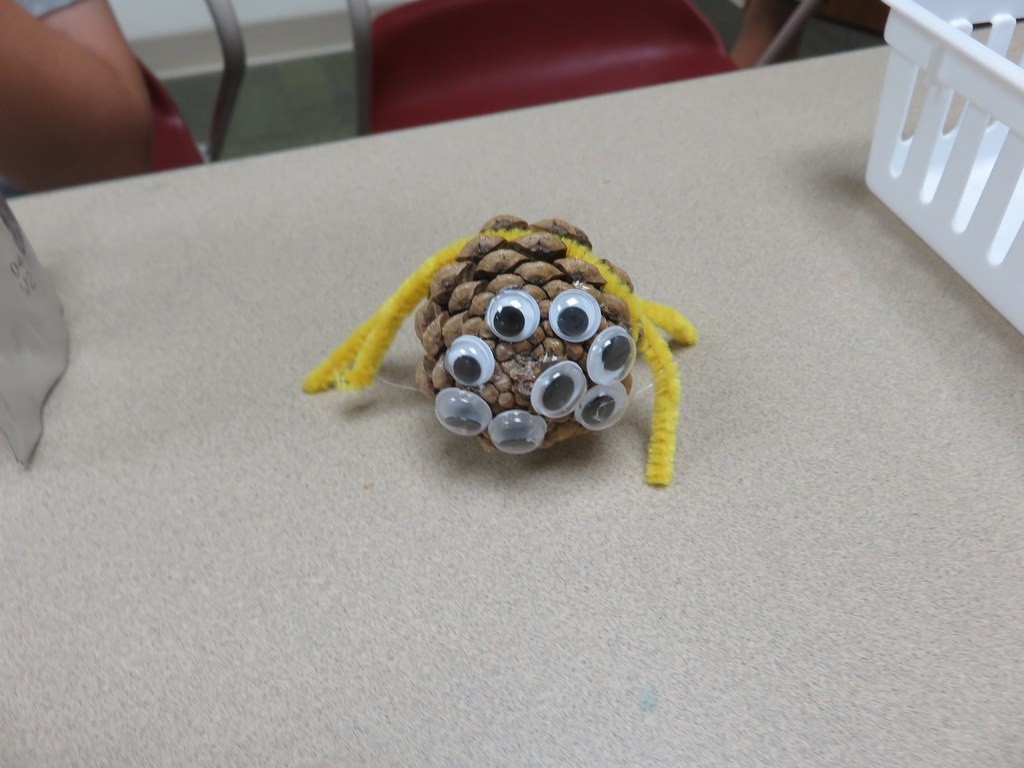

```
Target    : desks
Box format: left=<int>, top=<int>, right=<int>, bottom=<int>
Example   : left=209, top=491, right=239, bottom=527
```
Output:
left=0, top=19, right=1024, bottom=768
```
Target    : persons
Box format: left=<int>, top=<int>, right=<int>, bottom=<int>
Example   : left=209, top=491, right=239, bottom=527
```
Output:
left=0, top=0, right=157, bottom=196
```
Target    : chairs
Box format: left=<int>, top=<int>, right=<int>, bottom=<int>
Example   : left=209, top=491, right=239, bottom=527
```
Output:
left=128, top=0, right=825, bottom=173
